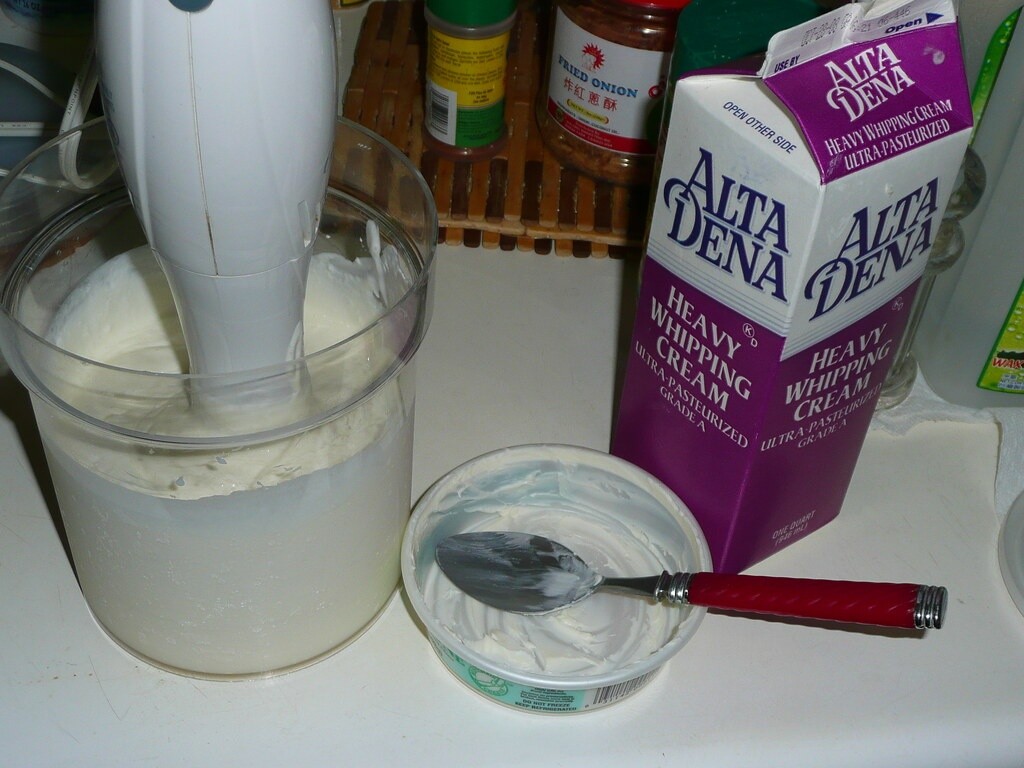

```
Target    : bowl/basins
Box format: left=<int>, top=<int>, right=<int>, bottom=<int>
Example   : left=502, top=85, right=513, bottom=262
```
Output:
left=400, top=444, right=713, bottom=714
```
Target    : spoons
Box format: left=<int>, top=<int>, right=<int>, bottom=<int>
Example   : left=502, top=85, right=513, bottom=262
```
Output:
left=434, top=531, right=949, bottom=631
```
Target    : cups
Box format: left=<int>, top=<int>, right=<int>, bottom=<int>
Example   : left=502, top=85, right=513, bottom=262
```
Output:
left=0, top=111, right=441, bottom=682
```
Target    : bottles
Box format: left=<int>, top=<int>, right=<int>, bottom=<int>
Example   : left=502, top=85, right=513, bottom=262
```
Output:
left=422, top=0, right=518, bottom=163
left=535, top=0, right=688, bottom=194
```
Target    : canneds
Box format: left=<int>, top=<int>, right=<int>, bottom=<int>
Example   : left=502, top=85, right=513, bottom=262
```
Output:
left=420, top=0, right=518, bottom=165
left=535, top=0, right=691, bottom=186
